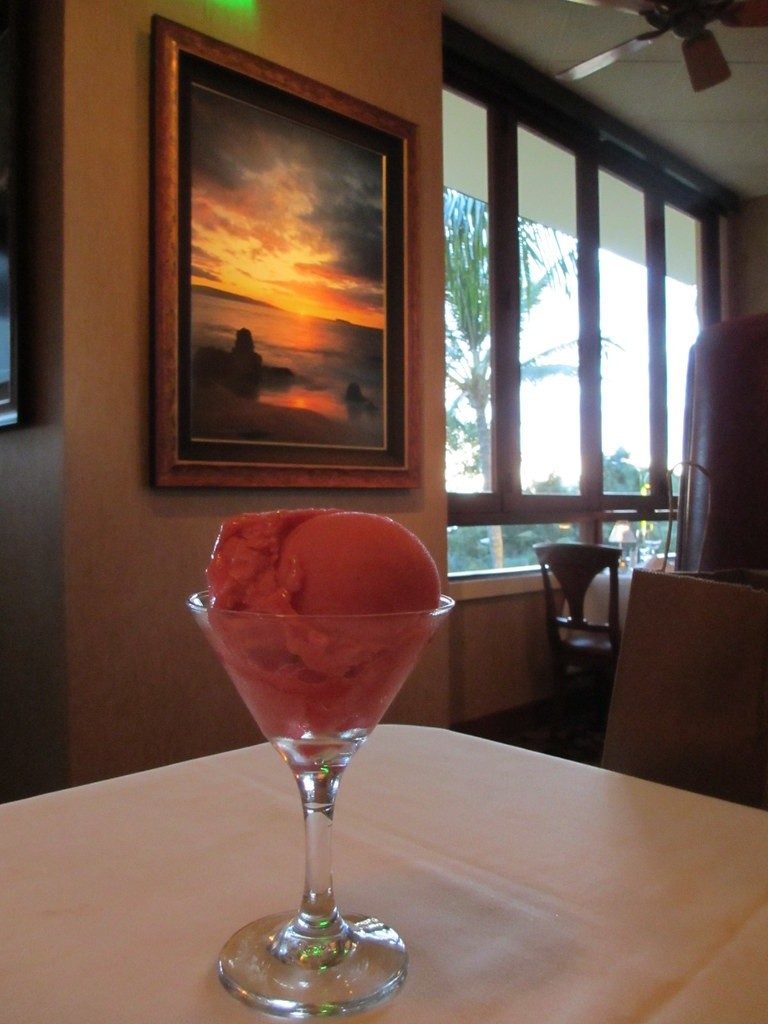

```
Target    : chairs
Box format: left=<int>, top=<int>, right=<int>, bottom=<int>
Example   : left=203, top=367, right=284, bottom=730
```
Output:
left=534, top=543, right=628, bottom=742
left=596, top=570, right=766, bottom=809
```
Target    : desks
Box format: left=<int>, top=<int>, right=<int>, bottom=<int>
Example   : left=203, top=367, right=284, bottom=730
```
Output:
left=3, top=722, right=768, bottom=1024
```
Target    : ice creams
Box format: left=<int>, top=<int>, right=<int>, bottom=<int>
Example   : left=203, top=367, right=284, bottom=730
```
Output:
left=205, top=506, right=441, bottom=745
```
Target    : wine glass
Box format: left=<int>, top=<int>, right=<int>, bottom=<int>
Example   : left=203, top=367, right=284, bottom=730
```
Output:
left=175, top=586, right=458, bottom=1016
left=636, top=529, right=661, bottom=561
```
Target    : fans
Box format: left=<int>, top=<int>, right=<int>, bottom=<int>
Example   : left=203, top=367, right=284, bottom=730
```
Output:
left=545, top=1, right=766, bottom=93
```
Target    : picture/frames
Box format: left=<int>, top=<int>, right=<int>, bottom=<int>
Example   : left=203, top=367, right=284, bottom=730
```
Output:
left=1, top=0, right=41, bottom=431
left=138, top=11, right=427, bottom=497
